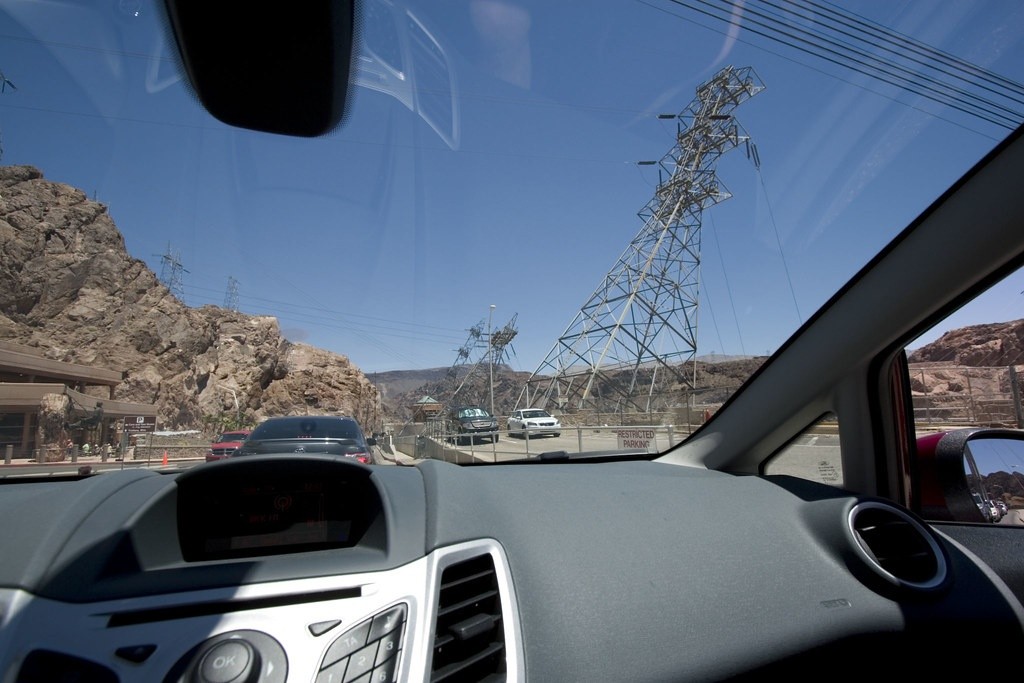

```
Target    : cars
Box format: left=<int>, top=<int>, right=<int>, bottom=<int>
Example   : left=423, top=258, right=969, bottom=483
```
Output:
left=506, top=408, right=561, bottom=439
left=232, top=416, right=377, bottom=465
left=206, top=430, right=251, bottom=462
left=971, top=494, right=993, bottom=523
left=985, top=499, right=1001, bottom=522
left=445, top=404, right=499, bottom=444
left=996, top=501, right=1008, bottom=516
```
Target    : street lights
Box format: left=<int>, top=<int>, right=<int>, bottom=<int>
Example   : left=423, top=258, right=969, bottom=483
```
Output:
left=489, top=304, right=496, bottom=415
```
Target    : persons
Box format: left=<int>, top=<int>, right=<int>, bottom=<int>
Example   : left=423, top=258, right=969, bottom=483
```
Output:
left=68, top=439, right=121, bottom=457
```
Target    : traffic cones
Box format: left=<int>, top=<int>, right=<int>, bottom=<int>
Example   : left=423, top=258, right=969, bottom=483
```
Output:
left=162, top=450, right=168, bottom=465
